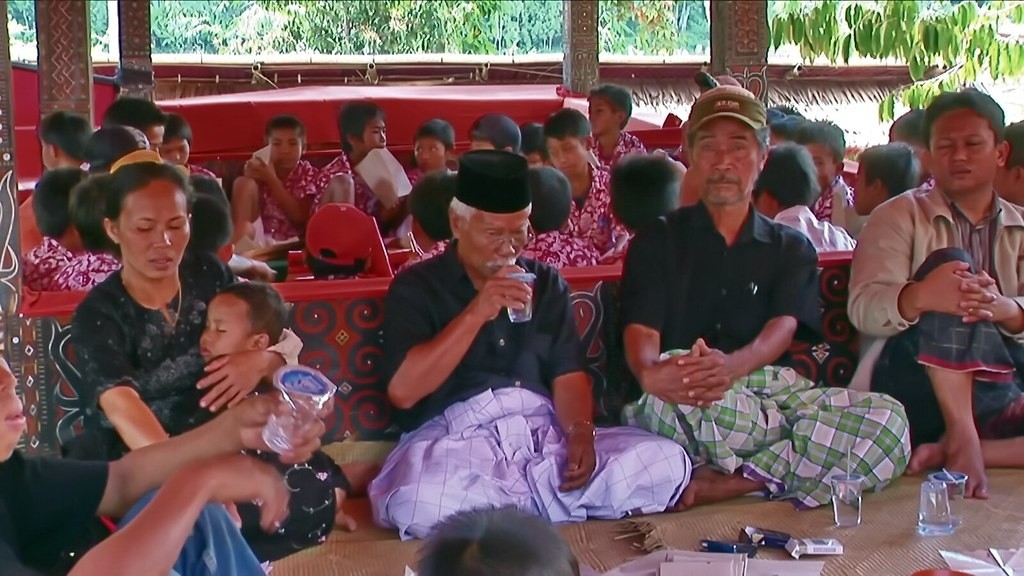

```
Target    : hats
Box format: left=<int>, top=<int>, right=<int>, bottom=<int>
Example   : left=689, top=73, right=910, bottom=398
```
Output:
left=687, top=84, right=767, bottom=134
left=454, top=148, right=532, bottom=213
left=305, top=203, right=372, bottom=265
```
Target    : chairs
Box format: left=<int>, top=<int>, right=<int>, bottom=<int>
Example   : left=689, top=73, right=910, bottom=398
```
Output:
left=306, top=203, right=392, bottom=275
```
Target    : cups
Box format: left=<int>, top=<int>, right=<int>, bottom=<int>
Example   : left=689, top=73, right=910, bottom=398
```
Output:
left=917, top=480, right=955, bottom=536
left=505, top=273, right=535, bottom=323
left=830, top=473, right=864, bottom=526
left=928, top=472, right=968, bottom=526
left=261, top=363, right=337, bottom=455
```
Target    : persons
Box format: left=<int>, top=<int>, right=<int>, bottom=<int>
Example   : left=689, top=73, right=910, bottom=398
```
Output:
left=401, top=502, right=583, bottom=576
left=69, top=160, right=358, bottom=563
left=60, top=281, right=289, bottom=463
left=620, top=84, right=914, bottom=512
left=18, top=72, right=1023, bottom=284
left=0, top=340, right=334, bottom=575
left=367, top=149, right=693, bottom=541
left=846, top=88, right=1024, bottom=499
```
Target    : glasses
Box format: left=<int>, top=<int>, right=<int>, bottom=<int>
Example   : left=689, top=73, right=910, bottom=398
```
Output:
left=462, top=216, right=536, bottom=247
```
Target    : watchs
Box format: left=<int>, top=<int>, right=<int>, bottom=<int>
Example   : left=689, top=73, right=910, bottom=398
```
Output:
left=563, top=418, right=597, bottom=439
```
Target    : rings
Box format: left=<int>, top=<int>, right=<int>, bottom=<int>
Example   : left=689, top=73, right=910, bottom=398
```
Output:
left=967, top=307, right=974, bottom=314
